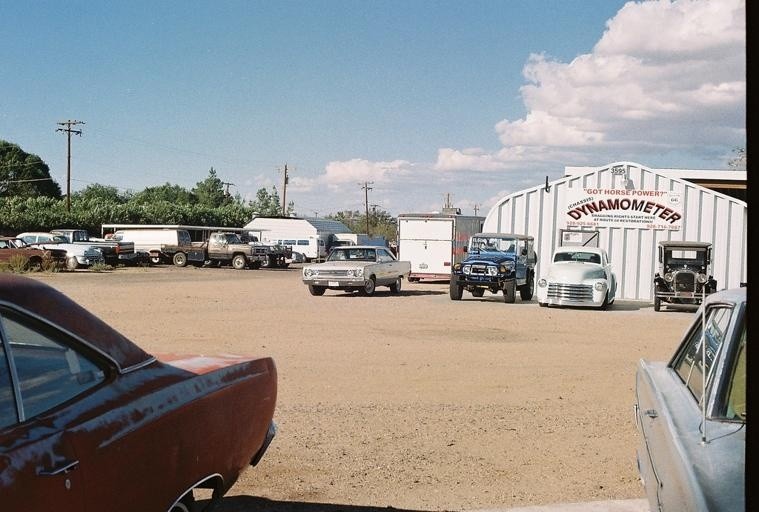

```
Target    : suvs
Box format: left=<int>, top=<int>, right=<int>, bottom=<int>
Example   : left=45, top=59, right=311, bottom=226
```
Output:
left=448, top=231, right=538, bottom=304
left=651, top=239, right=720, bottom=315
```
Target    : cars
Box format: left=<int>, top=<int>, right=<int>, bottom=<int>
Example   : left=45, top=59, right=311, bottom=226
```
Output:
left=0, top=268, right=286, bottom=511
left=534, top=244, right=620, bottom=310
left=301, top=243, right=414, bottom=297
left=629, top=279, right=748, bottom=512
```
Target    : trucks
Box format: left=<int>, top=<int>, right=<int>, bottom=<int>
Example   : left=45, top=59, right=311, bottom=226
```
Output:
left=1, top=223, right=296, bottom=273
left=396, top=212, right=487, bottom=284
